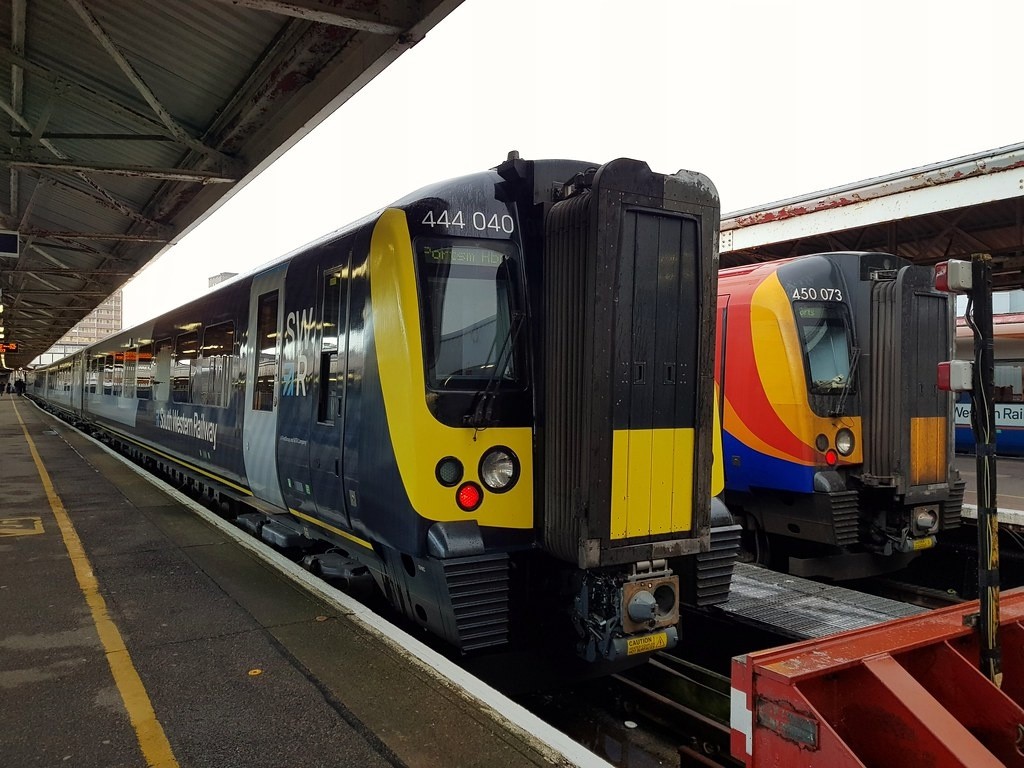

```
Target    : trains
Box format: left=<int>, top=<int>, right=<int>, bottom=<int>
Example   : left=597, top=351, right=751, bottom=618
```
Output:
left=954, top=310, right=1024, bottom=463
left=23, top=151, right=742, bottom=685
left=715, top=253, right=969, bottom=583
